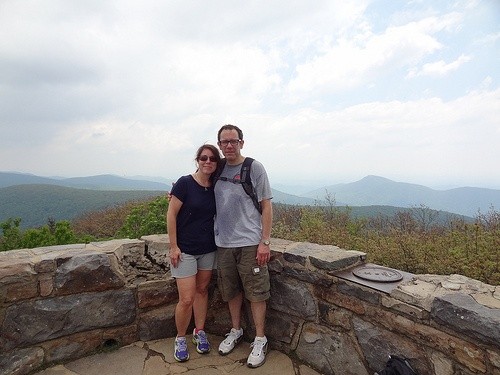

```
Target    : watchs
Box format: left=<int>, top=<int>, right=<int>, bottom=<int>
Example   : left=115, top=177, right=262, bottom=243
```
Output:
left=261, top=240, right=272, bottom=246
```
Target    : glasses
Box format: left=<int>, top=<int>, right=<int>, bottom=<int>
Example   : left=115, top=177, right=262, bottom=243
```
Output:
left=220, top=139, right=241, bottom=145
left=199, top=155, right=216, bottom=162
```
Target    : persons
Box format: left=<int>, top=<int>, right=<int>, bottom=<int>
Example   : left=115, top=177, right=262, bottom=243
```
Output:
left=167, top=125, right=272, bottom=368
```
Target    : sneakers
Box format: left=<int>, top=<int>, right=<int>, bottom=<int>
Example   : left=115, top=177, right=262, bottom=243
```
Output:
left=193, top=328, right=211, bottom=353
left=218, top=328, right=243, bottom=356
left=247, top=336, right=269, bottom=367
left=174, top=335, right=189, bottom=362
